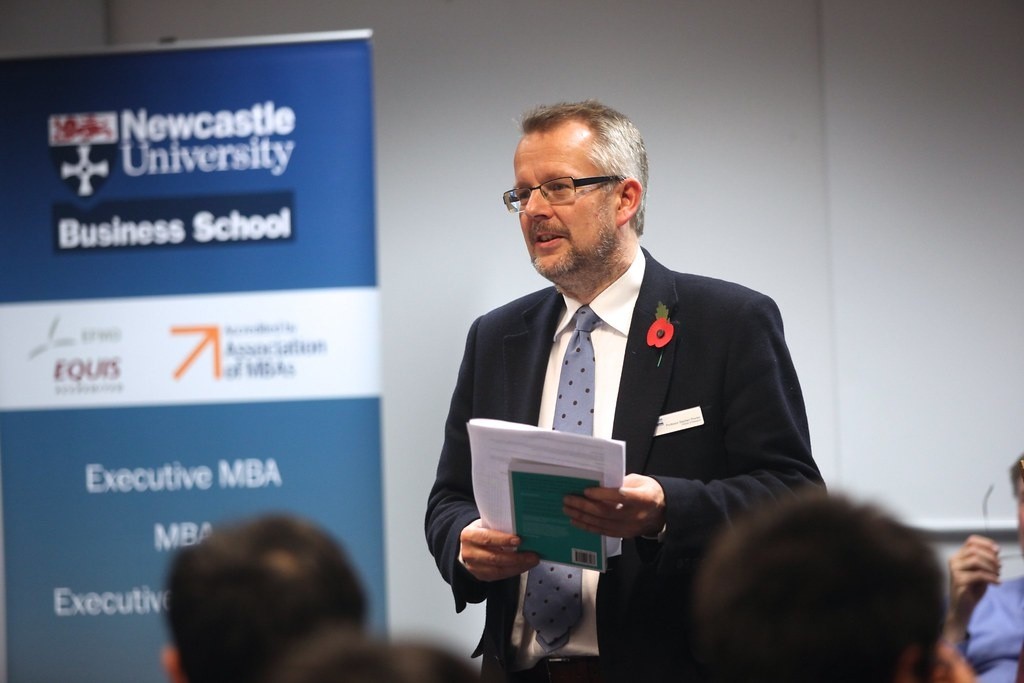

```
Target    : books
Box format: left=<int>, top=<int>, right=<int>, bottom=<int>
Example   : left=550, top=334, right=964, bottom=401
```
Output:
left=506, top=456, right=607, bottom=574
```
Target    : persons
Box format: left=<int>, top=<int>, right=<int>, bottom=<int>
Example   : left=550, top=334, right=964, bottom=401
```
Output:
left=423, top=99, right=829, bottom=683
left=942, top=453, right=1024, bottom=683
left=160, top=509, right=370, bottom=683
left=694, top=491, right=977, bottom=683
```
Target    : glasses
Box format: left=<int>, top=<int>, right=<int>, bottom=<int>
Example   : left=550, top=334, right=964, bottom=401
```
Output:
left=983, top=483, right=1024, bottom=574
left=502, top=174, right=627, bottom=214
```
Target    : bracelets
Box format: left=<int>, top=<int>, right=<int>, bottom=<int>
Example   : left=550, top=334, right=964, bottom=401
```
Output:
left=640, top=523, right=667, bottom=542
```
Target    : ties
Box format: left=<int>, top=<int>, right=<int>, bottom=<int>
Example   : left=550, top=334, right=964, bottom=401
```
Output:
left=523, top=305, right=602, bottom=655
